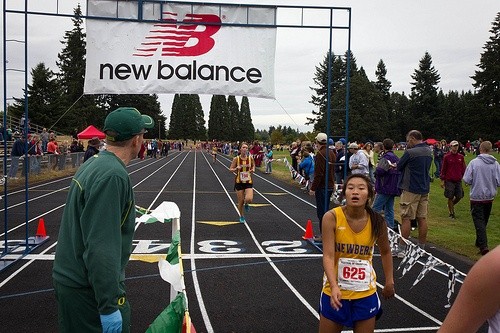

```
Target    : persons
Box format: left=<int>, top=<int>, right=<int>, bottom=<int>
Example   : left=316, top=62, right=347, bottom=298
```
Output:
left=371, top=138, right=396, bottom=236
left=360, top=141, right=375, bottom=191
left=434, top=244, right=499, bottom=333
left=230, top=143, right=256, bottom=224
left=310, top=133, right=337, bottom=242
left=347, top=143, right=372, bottom=178
left=430, top=138, right=484, bottom=179
left=136, top=138, right=349, bottom=193
left=51, top=107, right=155, bottom=332
left=84, top=137, right=104, bottom=166
left=440, top=141, right=468, bottom=218
left=395, top=131, right=434, bottom=258
left=317, top=174, right=395, bottom=333
left=0, top=112, right=91, bottom=183
left=373, top=143, right=385, bottom=183
left=462, top=141, right=499, bottom=256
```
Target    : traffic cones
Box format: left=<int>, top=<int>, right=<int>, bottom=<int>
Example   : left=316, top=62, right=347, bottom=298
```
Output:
left=34, top=217, right=47, bottom=240
left=302, top=220, right=316, bottom=240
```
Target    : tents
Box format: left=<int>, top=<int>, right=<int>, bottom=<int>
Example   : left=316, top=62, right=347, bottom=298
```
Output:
left=77, top=125, right=106, bottom=152
left=423, top=138, right=439, bottom=145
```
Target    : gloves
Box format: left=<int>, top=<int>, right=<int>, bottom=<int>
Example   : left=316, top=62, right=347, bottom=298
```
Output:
left=100, top=309, right=123, bottom=333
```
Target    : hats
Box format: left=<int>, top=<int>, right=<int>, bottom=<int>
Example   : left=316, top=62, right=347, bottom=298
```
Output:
left=347, top=143, right=358, bottom=148
left=88, top=137, right=103, bottom=147
left=315, top=132, right=327, bottom=143
left=449, top=140, right=459, bottom=145
left=103, top=106, right=155, bottom=142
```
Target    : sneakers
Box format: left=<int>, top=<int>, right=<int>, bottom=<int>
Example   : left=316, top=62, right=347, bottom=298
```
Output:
left=244, top=203, right=248, bottom=212
left=449, top=214, right=456, bottom=219
left=240, top=216, right=244, bottom=223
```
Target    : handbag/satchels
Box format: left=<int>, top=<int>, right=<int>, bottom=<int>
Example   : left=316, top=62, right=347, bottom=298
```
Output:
left=304, top=172, right=309, bottom=180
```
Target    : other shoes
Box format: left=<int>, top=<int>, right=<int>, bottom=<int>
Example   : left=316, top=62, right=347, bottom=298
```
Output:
left=301, top=187, right=307, bottom=190
left=475, top=244, right=489, bottom=255
left=313, top=234, right=322, bottom=241
left=411, top=226, right=415, bottom=231
left=265, top=172, right=272, bottom=174
left=9, top=177, right=19, bottom=179
